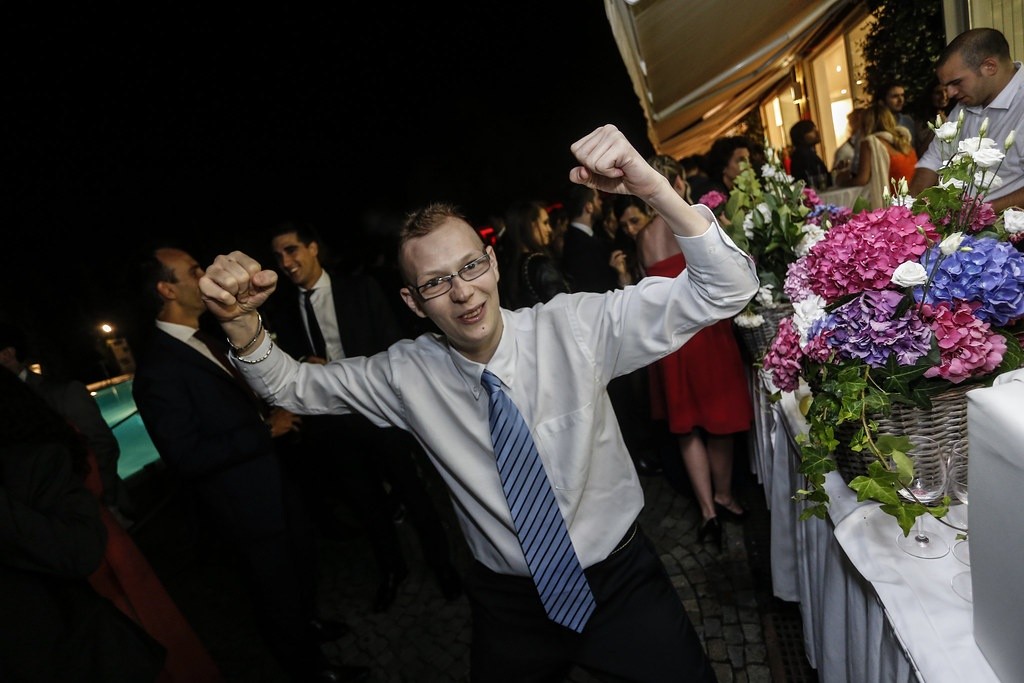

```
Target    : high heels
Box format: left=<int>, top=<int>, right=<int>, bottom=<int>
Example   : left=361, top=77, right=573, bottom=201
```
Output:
left=697, top=518, right=725, bottom=553
left=713, top=499, right=753, bottom=525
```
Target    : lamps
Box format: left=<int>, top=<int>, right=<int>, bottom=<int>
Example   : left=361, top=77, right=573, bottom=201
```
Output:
left=790, top=82, right=802, bottom=104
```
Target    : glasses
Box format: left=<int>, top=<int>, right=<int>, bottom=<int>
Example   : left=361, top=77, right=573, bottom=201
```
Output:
left=408, top=252, right=491, bottom=299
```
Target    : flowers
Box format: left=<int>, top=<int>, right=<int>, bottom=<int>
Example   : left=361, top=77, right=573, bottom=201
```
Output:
left=694, top=107, right=1024, bottom=541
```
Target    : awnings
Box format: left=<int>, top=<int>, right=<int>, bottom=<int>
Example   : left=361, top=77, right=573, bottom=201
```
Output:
left=605, top=0, right=886, bottom=162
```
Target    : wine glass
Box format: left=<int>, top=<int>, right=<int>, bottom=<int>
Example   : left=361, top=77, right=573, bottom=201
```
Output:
left=889, top=433, right=950, bottom=559
left=946, top=437, right=971, bottom=567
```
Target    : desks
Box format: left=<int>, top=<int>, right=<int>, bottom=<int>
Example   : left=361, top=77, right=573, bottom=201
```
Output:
left=746, top=350, right=1002, bottom=683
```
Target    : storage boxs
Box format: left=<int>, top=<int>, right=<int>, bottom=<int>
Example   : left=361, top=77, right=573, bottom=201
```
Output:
left=964, top=366, right=1024, bottom=683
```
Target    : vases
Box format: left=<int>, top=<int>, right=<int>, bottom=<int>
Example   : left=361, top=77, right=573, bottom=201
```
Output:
left=836, top=369, right=989, bottom=506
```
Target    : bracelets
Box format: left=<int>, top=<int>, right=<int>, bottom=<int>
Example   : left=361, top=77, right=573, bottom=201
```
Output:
left=236, top=330, right=277, bottom=364
left=226, top=311, right=263, bottom=353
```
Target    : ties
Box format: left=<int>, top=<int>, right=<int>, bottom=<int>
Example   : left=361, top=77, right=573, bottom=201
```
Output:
left=480, top=371, right=596, bottom=632
left=194, top=330, right=238, bottom=377
left=301, top=289, right=327, bottom=360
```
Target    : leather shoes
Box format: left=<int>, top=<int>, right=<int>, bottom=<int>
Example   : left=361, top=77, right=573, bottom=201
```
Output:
left=433, top=566, right=463, bottom=601
left=636, top=459, right=668, bottom=477
left=322, top=663, right=370, bottom=683
left=370, top=566, right=410, bottom=614
left=309, top=617, right=351, bottom=643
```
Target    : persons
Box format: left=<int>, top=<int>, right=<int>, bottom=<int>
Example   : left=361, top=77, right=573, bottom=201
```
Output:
left=199, top=123, right=762, bottom=683
left=0, top=324, right=220, bottom=683
left=269, top=213, right=464, bottom=605
left=635, top=153, right=751, bottom=543
left=132, top=246, right=373, bottom=683
left=477, top=119, right=829, bottom=310
left=906, top=27, right=1024, bottom=253
left=831, top=77, right=961, bottom=211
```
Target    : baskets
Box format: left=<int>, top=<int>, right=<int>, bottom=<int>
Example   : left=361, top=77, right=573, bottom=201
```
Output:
left=738, top=304, right=795, bottom=365
left=808, top=380, right=986, bottom=507
